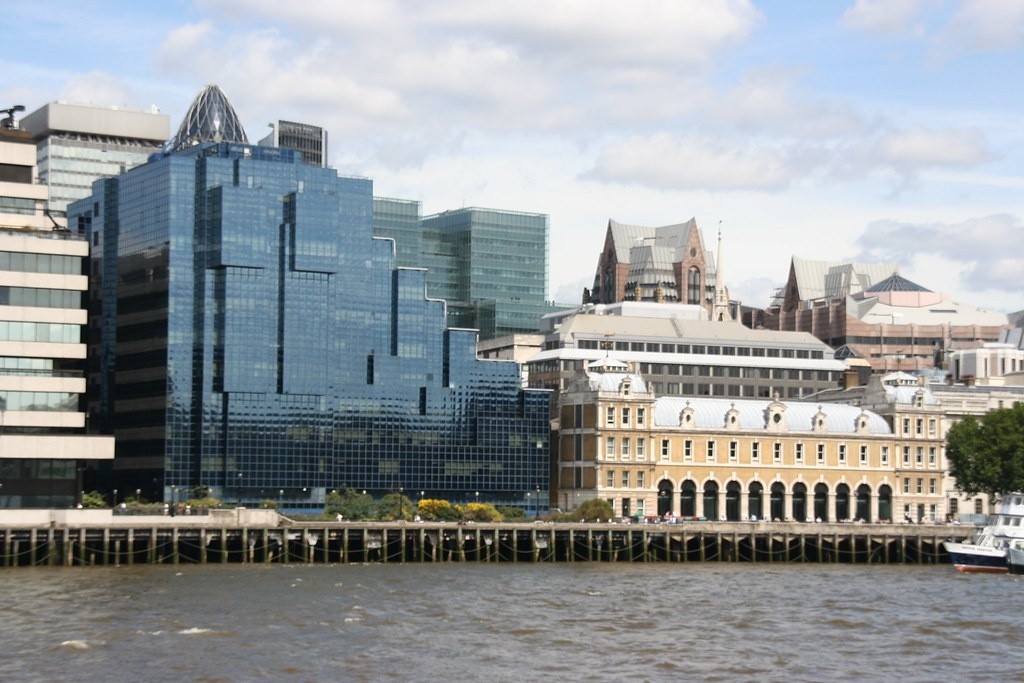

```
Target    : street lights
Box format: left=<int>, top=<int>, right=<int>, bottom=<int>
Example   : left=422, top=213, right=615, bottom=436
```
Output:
left=171, top=484, right=175, bottom=507
left=114, top=489, right=117, bottom=505
left=475, top=491, right=480, bottom=504
left=238, top=472, right=244, bottom=507
left=535, top=485, right=541, bottom=521
left=136, top=488, right=141, bottom=502
left=399, top=487, right=404, bottom=516
left=278, top=488, right=283, bottom=514
left=526, top=491, right=531, bottom=520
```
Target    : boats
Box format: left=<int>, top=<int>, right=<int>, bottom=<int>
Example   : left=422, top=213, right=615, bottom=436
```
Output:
left=1004, top=546, right=1024, bottom=577
left=942, top=491, right=1024, bottom=574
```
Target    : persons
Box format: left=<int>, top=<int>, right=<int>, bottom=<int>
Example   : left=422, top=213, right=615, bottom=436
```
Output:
left=76, top=502, right=191, bottom=517
left=336, top=511, right=960, bottom=525
